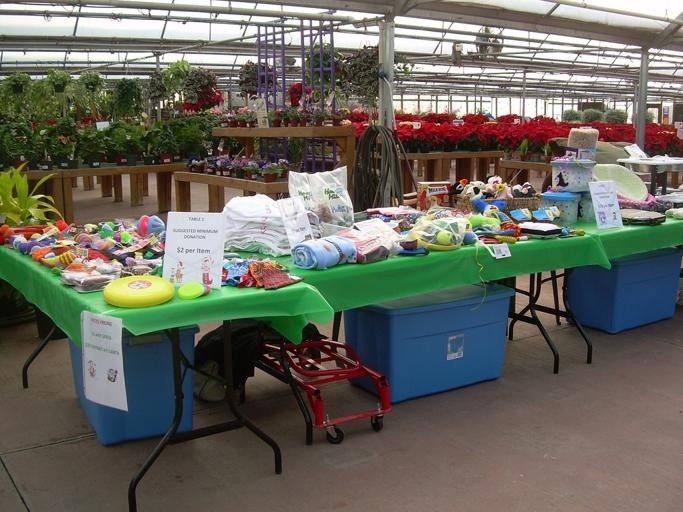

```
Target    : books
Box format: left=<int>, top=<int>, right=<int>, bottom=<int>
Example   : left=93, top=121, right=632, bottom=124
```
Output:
left=415, top=179, right=453, bottom=213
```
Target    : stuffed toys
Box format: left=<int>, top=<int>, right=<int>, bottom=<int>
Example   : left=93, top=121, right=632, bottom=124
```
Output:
left=0, top=213, right=168, bottom=278
left=450, top=176, right=538, bottom=201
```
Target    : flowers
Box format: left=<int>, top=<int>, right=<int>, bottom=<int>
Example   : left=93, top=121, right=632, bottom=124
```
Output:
left=351, top=111, right=683, bottom=154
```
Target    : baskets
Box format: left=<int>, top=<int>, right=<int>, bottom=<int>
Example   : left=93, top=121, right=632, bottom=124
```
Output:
left=456, top=191, right=540, bottom=224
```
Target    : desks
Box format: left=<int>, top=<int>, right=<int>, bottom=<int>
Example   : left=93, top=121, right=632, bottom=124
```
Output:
left=0, top=225, right=302, bottom=510
left=222, top=233, right=594, bottom=450
left=504, top=156, right=682, bottom=364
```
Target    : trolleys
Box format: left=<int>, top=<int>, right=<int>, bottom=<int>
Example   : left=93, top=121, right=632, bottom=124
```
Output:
left=255, top=339, right=392, bottom=445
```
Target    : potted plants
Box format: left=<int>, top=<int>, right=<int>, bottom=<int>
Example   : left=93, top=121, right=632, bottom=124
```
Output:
left=0, top=57, right=343, bottom=181
